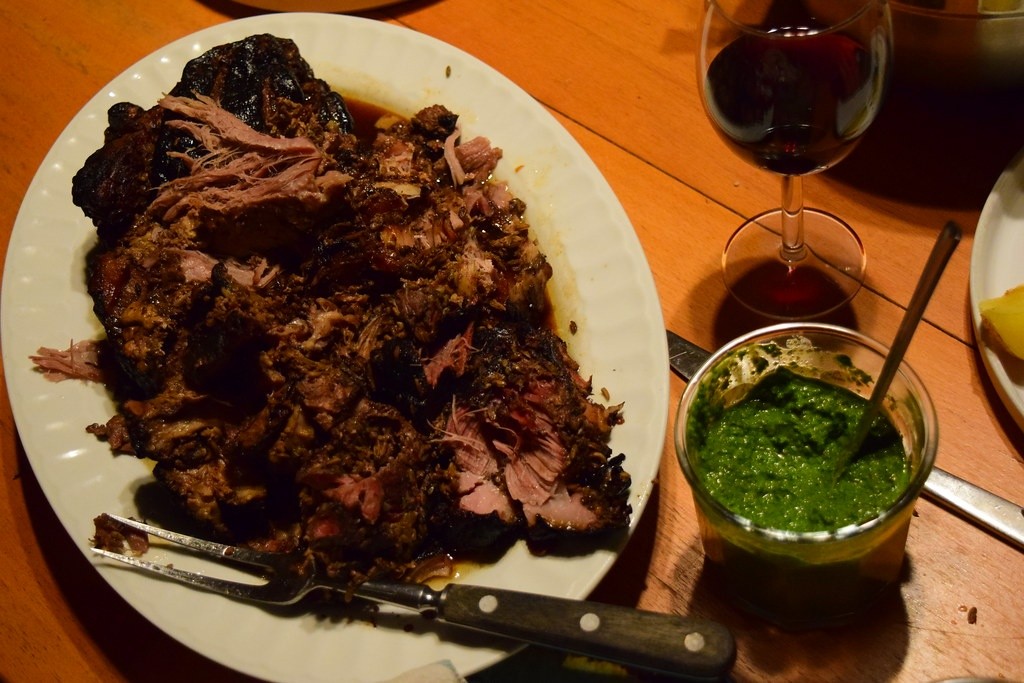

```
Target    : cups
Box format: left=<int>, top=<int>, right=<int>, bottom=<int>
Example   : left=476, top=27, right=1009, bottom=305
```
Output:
left=672, top=321, right=940, bottom=597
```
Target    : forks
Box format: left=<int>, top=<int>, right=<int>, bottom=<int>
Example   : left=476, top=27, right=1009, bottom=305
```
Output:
left=88, top=510, right=737, bottom=683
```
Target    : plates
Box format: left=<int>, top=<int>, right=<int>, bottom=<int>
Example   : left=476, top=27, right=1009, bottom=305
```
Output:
left=968, top=158, right=1024, bottom=432
left=0, top=8, right=670, bottom=682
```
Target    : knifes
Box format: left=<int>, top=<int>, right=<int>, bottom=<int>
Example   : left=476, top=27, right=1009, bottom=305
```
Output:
left=666, top=328, right=1024, bottom=552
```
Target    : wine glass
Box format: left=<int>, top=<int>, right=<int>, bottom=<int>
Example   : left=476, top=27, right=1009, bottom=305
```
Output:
left=692, top=0, right=895, bottom=320
